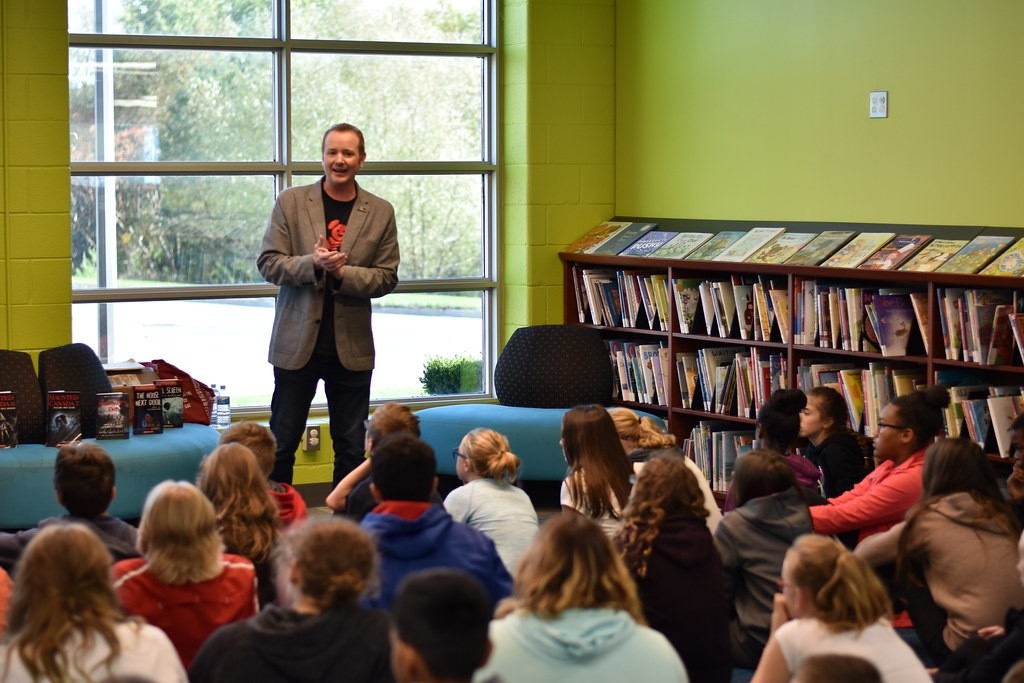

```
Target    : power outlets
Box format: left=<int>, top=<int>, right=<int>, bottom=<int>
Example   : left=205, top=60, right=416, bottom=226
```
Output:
left=303, top=425, right=320, bottom=452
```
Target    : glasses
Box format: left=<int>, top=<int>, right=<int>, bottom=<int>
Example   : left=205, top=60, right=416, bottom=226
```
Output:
left=363, top=419, right=371, bottom=432
left=453, top=448, right=474, bottom=472
left=877, top=420, right=919, bottom=436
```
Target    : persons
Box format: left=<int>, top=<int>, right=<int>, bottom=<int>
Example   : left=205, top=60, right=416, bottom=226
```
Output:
left=257, top=122, right=400, bottom=517
left=0, top=383, right=1024, bottom=683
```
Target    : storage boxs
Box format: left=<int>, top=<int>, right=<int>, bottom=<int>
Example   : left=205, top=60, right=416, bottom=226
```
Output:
left=133, top=385, right=163, bottom=435
left=96, top=392, right=130, bottom=440
left=46, top=390, right=80, bottom=447
left=152, top=379, right=184, bottom=429
left=0, top=391, right=18, bottom=449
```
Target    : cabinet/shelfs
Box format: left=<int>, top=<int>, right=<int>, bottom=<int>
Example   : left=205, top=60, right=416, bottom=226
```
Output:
left=559, top=216, right=1024, bottom=515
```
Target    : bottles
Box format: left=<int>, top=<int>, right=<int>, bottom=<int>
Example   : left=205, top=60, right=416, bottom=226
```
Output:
left=209, top=384, right=232, bottom=430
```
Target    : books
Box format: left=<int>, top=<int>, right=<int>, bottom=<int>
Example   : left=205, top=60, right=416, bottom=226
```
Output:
left=0, top=391, right=19, bottom=449
left=154, top=379, right=183, bottom=429
left=132, top=385, right=163, bottom=434
left=45, top=391, right=81, bottom=447
left=567, top=220, right=1024, bottom=492
left=102, top=358, right=144, bottom=375
left=96, top=392, right=129, bottom=439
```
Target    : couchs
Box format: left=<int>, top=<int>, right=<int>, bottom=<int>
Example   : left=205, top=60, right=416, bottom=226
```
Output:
left=413, top=324, right=666, bottom=510
left=0, top=343, right=221, bottom=534
